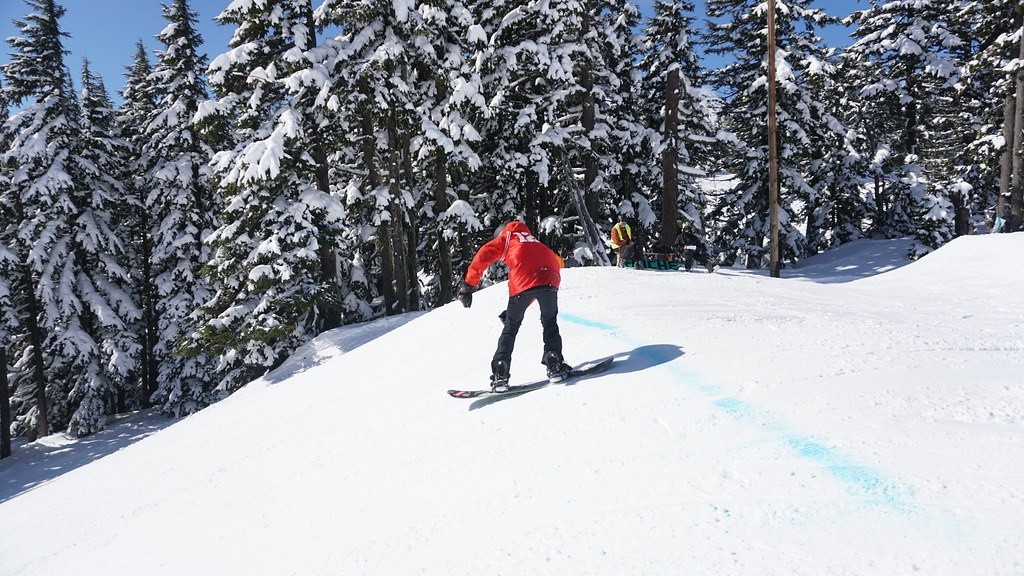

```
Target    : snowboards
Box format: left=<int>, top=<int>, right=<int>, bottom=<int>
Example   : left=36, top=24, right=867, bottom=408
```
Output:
left=446, top=356, right=617, bottom=400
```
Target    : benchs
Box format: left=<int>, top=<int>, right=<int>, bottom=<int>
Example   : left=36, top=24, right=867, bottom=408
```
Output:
left=614, top=251, right=665, bottom=270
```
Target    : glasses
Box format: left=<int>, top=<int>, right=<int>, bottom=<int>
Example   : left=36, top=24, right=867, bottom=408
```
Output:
left=619, top=220, right=626, bottom=222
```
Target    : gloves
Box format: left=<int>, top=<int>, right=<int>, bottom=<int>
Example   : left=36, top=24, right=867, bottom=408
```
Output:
left=499, top=309, right=507, bottom=324
left=457, top=280, right=475, bottom=308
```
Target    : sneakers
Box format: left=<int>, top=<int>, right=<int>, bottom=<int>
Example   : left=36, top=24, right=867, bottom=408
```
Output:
left=489, top=360, right=511, bottom=392
left=543, top=351, right=573, bottom=383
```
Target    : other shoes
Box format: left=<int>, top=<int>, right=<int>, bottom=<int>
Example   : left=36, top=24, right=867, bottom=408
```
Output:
left=706, top=262, right=716, bottom=272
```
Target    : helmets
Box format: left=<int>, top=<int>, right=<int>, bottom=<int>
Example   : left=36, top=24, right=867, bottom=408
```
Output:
left=494, top=222, right=508, bottom=239
left=618, top=215, right=626, bottom=221
left=682, top=222, right=692, bottom=231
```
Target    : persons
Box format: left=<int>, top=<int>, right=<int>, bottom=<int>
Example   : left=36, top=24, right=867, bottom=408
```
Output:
left=612, top=215, right=645, bottom=271
left=674, top=223, right=718, bottom=273
left=458, top=220, right=570, bottom=393
